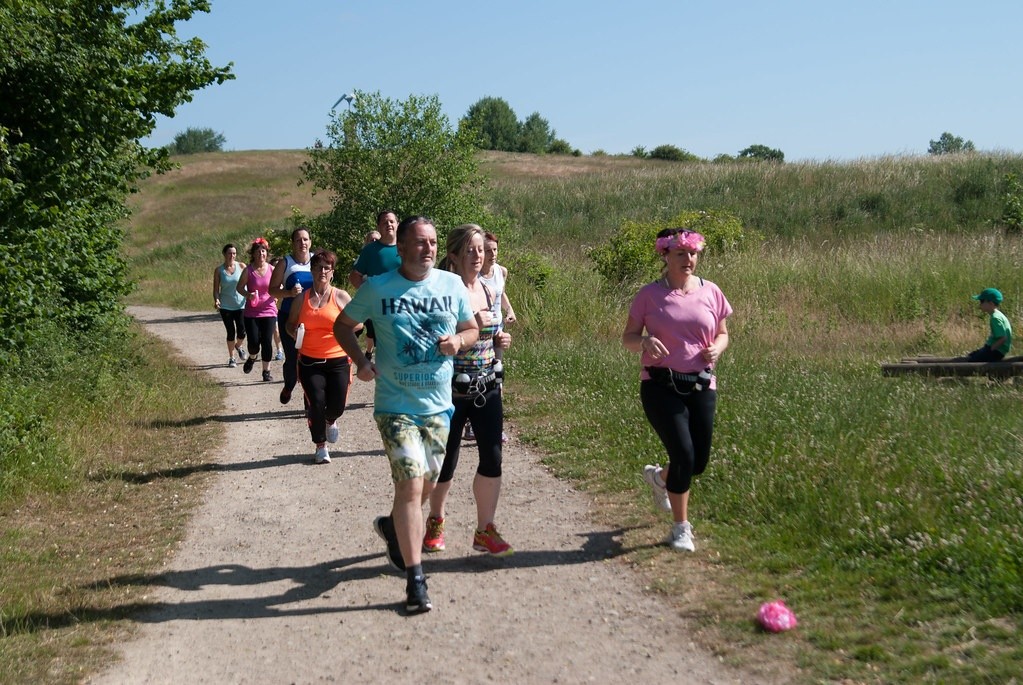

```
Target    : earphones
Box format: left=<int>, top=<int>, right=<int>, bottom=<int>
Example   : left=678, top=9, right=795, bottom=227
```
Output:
left=481, top=372, right=488, bottom=377
left=666, top=256, right=669, bottom=263
left=478, top=376, right=483, bottom=379
left=332, top=272, right=333, bottom=278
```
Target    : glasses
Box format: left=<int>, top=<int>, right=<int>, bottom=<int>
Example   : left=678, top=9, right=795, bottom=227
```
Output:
left=311, top=265, right=333, bottom=272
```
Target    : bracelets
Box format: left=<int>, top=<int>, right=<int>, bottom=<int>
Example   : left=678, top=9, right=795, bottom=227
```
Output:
left=458, top=333, right=467, bottom=348
left=639, top=336, right=650, bottom=352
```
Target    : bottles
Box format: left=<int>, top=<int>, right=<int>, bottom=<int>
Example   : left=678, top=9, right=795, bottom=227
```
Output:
left=455, top=369, right=470, bottom=382
left=251, top=290, right=259, bottom=307
left=493, top=359, right=503, bottom=383
left=695, top=366, right=711, bottom=390
left=295, top=279, right=302, bottom=293
left=294, top=322, right=305, bottom=349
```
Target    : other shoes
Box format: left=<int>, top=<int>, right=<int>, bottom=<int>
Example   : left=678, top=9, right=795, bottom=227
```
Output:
left=262, top=370, right=272, bottom=381
left=464, top=421, right=475, bottom=440
left=229, top=358, right=236, bottom=368
left=280, top=386, right=292, bottom=404
left=243, top=355, right=257, bottom=374
left=501, top=431, right=508, bottom=445
left=235, top=344, right=247, bottom=361
left=275, top=352, right=282, bottom=360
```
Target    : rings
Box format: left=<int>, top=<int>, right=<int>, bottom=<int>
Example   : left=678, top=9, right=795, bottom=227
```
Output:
left=655, top=352, right=659, bottom=356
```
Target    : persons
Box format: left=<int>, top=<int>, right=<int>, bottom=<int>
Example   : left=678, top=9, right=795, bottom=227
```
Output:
left=211, top=238, right=284, bottom=381
left=966, top=288, right=1012, bottom=362
left=621, top=226, right=734, bottom=553
left=478, top=231, right=517, bottom=443
left=267, top=229, right=315, bottom=405
left=285, top=248, right=364, bottom=464
left=363, top=230, right=381, bottom=361
left=348, top=210, right=401, bottom=348
left=333, top=216, right=478, bottom=615
left=423, top=222, right=513, bottom=558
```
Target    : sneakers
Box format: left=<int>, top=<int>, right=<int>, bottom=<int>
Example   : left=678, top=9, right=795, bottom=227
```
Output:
left=421, top=516, right=445, bottom=552
left=668, top=521, right=696, bottom=552
left=406, top=575, right=431, bottom=613
left=316, top=446, right=331, bottom=464
left=644, top=463, right=671, bottom=508
left=473, top=522, right=512, bottom=558
left=326, top=420, right=338, bottom=443
left=373, top=515, right=405, bottom=571
